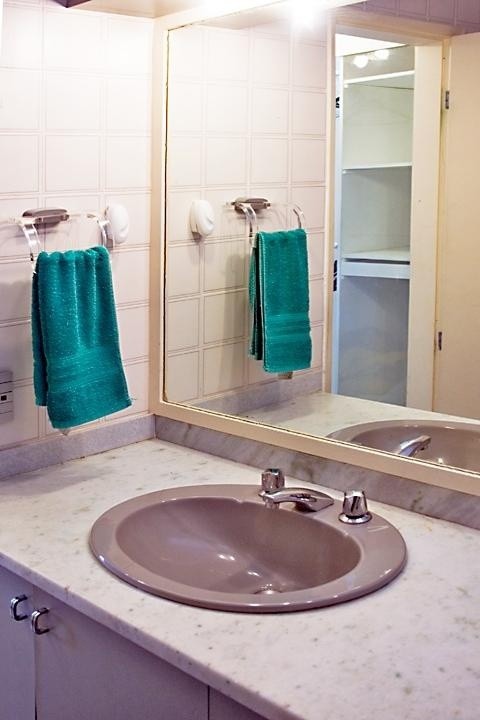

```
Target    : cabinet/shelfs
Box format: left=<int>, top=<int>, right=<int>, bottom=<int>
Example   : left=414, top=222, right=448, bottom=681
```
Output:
left=1, top=559, right=210, bottom=718
left=331, top=40, right=422, bottom=399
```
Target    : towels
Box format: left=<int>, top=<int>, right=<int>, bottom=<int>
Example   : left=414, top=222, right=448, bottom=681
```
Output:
left=30, top=250, right=136, bottom=434
left=247, top=226, right=316, bottom=377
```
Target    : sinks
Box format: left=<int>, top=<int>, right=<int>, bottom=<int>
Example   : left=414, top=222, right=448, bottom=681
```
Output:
left=89, top=483, right=407, bottom=612
left=327, top=419, right=480, bottom=473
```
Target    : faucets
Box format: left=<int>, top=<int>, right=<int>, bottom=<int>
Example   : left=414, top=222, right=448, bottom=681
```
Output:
left=262, top=487, right=335, bottom=512
left=391, top=434, right=432, bottom=458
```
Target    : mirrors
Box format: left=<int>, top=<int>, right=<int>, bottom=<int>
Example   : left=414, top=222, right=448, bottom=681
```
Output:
left=150, top=6, right=479, bottom=494
